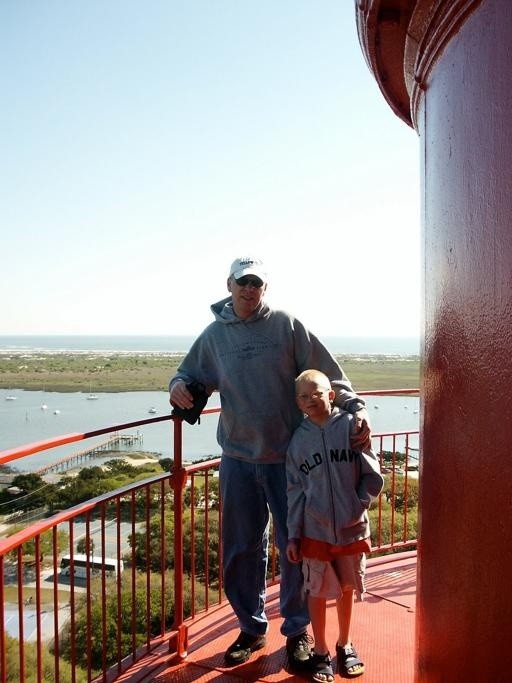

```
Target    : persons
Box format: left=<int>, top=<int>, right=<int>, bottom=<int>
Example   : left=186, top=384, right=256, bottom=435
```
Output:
left=287, top=369, right=385, bottom=682
left=168, top=253, right=371, bottom=669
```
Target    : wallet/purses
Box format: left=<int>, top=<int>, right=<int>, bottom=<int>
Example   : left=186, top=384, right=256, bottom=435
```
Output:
left=174, top=383, right=208, bottom=425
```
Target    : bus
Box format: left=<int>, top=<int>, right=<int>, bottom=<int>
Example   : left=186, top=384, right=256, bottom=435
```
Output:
left=60, top=554, right=124, bottom=581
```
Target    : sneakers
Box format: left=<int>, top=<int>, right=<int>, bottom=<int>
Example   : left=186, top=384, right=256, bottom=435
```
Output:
left=223, top=631, right=266, bottom=666
left=286, top=631, right=313, bottom=673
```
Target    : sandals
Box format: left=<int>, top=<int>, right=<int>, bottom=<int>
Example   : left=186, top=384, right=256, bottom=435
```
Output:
left=311, top=647, right=335, bottom=683
left=336, top=642, right=365, bottom=675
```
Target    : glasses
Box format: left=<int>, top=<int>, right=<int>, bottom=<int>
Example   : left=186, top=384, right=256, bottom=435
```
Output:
left=236, top=277, right=263, bottom=288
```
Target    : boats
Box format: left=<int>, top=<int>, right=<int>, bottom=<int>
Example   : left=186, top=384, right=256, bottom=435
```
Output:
left=147, top=406, right=156, bottom=413
left=5, top=394, right=99, bottom=416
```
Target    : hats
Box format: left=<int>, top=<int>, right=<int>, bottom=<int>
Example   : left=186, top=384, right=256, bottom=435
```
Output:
left=228, top=257, right=268, bottom=283
